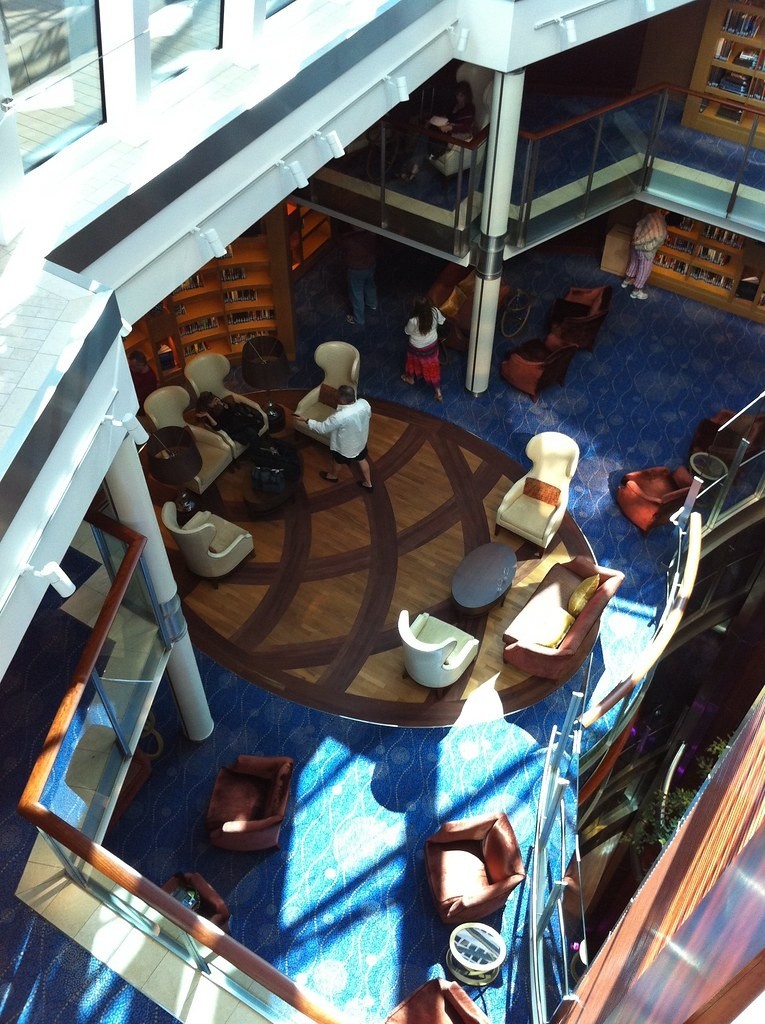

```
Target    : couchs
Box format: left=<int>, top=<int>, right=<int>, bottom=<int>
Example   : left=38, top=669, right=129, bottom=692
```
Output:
left=689, top=409, right=765, bottom=471
left=161, top=871, right=231, bottom=935
left=426, top=262, right=511, bottom=352
left=546, top=283, right=615, bottom=351
left=616, top=465, right=704, bottom=532
left=107, top=746, right=152, bottom=831
left=206, top=755, right=294, bottom=851
left=384, top=978, right=492, bottom=1024
left=503, top=556, right=626, bottom=686
left=500, top=338, right=580, bottom=403
left=424, top=812, right=526, bottom=926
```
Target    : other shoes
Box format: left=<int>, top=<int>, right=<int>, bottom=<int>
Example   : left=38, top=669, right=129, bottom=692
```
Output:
left=357, top=480, right=373, bottom=490
left=346, top=315, right=356, bottom=325
left=366, top=302, right=376, bottom=310
left=434, top=393, right=442, bottom=402
left=401, top=375, right=414, bottom=386
left=320, top=471, right=338, bottom=483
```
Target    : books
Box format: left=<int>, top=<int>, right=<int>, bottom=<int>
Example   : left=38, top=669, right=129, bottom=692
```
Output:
left=224, top=289, right=258, bottom=302
left=652, top=215, right=742, bottom=290
left=175, top=302, right=184, bottom=314
left=707, top=9, right=765, bottom=101
left=227, top=309, right=275, bottom=325
left=171, top=274, right=205, bottom=296
left=158, top=345, right=175, bottom=369
left=184, top=341, right=210, bottom=356
left=232, top=332, right=269, bottom=344
left=222, top=268, right=244, bottom=281
left=179, top=317, right=219, bottom=335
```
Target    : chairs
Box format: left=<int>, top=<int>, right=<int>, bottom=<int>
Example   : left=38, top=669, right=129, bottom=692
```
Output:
left=161, top=502, right=254, bottom=590
left=183, top=353, right=269, bottom=458
left=292, top=340, right=361, bottom=449
left=427, top=63, right=496, bottom=177
left=495, top=431, right=580, bottom=558
left=143, top=385, right=232, bottom=496
left=397, top=610, right=480, bottom=700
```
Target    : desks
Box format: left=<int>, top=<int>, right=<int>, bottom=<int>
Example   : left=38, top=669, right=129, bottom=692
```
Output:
left=600, top=223, right=633, bottom=276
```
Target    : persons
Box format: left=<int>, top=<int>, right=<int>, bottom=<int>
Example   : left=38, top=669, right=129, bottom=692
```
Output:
left=195, top=391, right=256, bottom=457
left=400, top=295, right=447, bottom=403
left=295, top=385, right=373, bottom=488
left=621, top=208, right=669, bottom=300
left=428, top=80, right=476, bottom=160
left=342, top=223, right=377, bottom=325
left=129, top=351, right=158, bottom=416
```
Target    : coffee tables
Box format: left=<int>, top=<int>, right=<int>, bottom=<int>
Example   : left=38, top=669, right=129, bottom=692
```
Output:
left=690, top=452, right=728, bottom=483
left=451, top=541, right=517, bottom=621
left=447, top=921, right=507, bottom=988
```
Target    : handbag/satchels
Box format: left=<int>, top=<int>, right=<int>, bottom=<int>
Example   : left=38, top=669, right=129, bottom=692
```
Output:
left=433, top=307, right=450, bottom=338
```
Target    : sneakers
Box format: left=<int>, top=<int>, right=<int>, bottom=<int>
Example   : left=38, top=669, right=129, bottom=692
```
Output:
left=621, top=277, right=635, bottom=288
left=630, top=290, right=648, bottom=299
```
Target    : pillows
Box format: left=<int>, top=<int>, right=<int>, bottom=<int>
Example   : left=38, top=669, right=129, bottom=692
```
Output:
left=528, top=606, right=575, bottom=648
left=439, top=286, right=466, bottom=318
left=456, top=269, right=476, bottom=296
left=567, top=574, right=600, bottom=617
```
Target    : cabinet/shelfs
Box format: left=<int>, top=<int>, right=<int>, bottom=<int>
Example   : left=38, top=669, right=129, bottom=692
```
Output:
left=125, top=199, right=292, bottom=400
left=680, top=0, right=765, bottom=150
left=647, top=209, right=765, bottom=324
